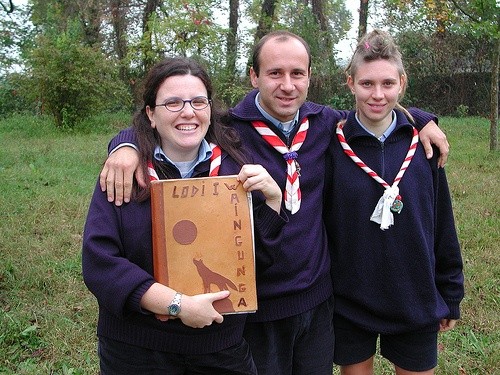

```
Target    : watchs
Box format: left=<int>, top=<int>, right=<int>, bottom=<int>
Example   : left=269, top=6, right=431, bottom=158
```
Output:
left=168, top=292, right=182, bottom=317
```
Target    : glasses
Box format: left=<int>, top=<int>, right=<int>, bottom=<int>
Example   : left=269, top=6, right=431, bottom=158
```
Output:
left=155, top=96, right=213, bottom=112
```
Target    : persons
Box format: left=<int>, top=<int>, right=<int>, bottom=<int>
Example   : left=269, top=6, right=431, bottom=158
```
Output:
left=321, top=30, right=465, bottom=375
left=82, top=56, right=290, bottom=375
left=99, top=30, right=450, bottom=375
